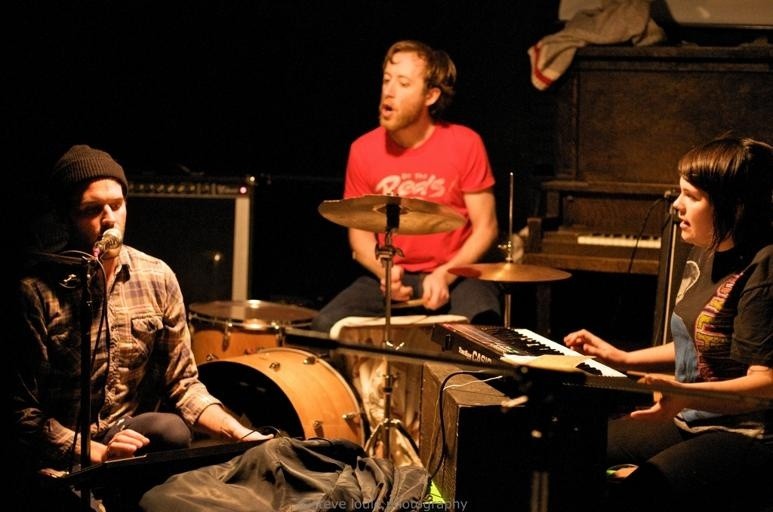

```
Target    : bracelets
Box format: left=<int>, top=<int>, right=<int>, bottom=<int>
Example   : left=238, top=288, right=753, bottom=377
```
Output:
left=233, top=427, right=258, bottom=442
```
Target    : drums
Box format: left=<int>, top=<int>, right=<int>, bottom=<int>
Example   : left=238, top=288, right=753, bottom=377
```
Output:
left=191, top=298, right=321, bottom=363
left=197, top=346, right=365, bottom=453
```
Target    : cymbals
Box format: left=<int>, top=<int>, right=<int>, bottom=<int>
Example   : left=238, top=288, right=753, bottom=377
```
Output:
left=448, top=262, right=572, bottom=282
left=319, top=196, right=467, bottom=234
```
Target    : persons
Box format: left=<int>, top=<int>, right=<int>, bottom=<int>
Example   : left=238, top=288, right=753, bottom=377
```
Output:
left=1, top=145, right=277, bottom=511
left=310, top=40, right=502, bottom=333
left=562, top=132, right=772, bottom=509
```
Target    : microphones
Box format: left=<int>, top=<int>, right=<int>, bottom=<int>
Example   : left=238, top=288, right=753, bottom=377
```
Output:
left=91, top=227, right=124, bottom=269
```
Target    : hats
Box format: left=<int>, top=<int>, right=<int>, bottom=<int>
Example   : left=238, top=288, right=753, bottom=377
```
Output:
left=47, top=141, right=129, bottom=204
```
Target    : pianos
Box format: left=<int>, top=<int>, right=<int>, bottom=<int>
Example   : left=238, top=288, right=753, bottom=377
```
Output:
left=519, top=42, right=772, bottom=342
left=432, top=323, right=653, bottom=409
